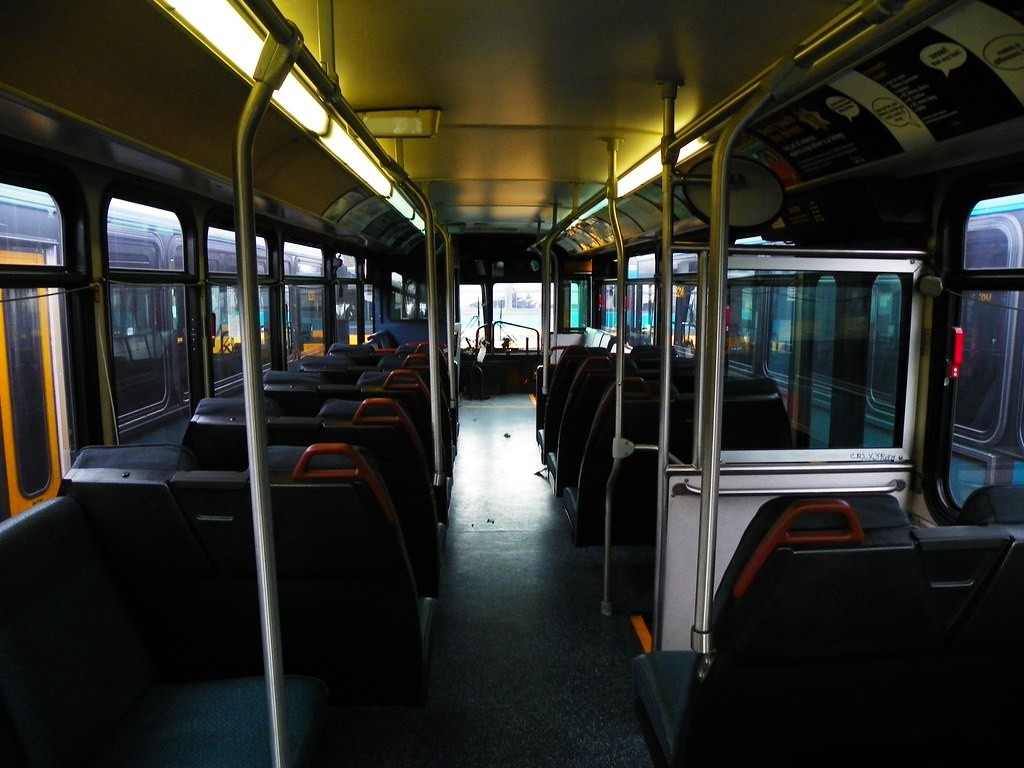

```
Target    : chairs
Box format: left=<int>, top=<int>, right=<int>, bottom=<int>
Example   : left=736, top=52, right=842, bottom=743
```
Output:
left=0, top=339, right=461, bottom=768
left=534, top=341, right=1024, bottom=768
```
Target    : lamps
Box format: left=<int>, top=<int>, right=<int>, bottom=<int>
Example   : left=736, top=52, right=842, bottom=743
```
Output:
left=558, top=0, right=934, bottom=233
left=149, top=0, right=427, bottom=234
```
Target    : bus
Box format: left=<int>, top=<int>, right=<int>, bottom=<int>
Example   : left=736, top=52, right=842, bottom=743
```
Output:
left=0, top=182, right=373, bottom=525
left=579, top=194, right=1024, bottom=509
